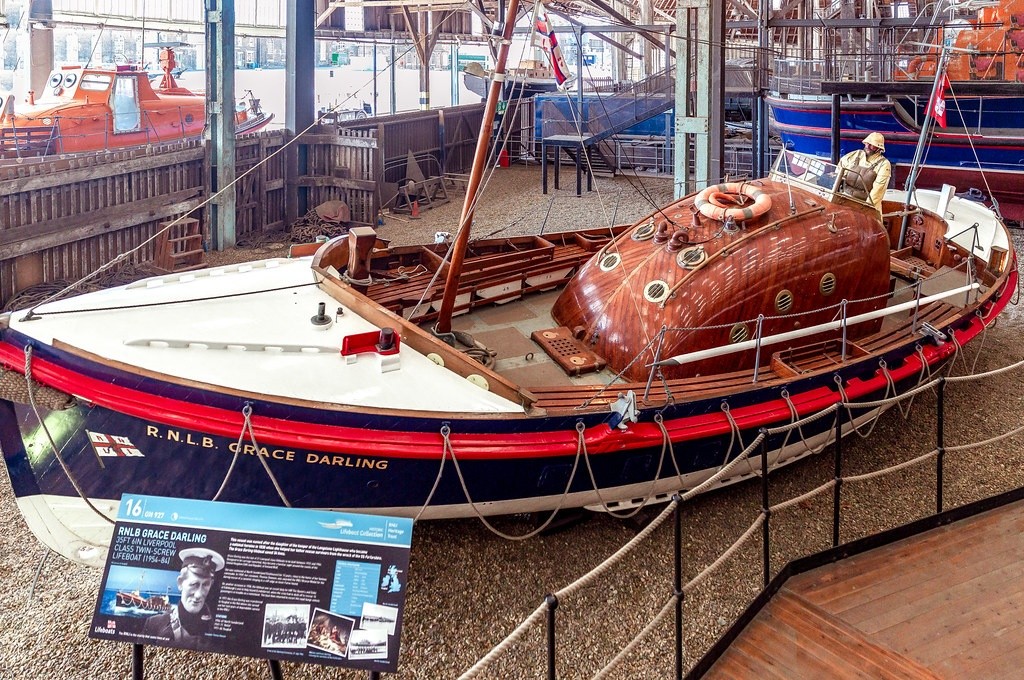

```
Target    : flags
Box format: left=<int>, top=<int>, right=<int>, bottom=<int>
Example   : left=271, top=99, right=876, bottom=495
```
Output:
left=530, top=2, right=573, bottom=93
left=923, top=59, right=949, bottom=128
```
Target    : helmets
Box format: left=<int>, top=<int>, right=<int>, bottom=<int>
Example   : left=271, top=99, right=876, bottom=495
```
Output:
left=862, top=132, right=885, bottom=154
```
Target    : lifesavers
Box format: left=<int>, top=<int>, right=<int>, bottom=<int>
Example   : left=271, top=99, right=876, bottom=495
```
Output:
left=693, top=182, right=771, bottom=222
left=907, top=54, right=945, bottom=82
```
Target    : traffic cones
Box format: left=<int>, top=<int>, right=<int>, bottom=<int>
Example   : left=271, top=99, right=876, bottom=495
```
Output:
left=411, top=200, right=420, bottom=217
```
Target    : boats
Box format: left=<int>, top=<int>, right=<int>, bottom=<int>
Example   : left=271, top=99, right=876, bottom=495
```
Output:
left=0, top=41, right=278, bottom=180
left=1, top=181, right=1020, bottom=570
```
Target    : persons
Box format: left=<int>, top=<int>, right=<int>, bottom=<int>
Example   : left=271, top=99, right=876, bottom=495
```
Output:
left=835, top=132, right=891, bottom=224
left=264, top=615, right=306, bottom=645
left=141, top=548, right=225, bottom=640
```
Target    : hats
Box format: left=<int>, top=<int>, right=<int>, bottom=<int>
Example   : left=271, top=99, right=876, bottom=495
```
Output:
left=178, top=547, right=225, bottom=578
